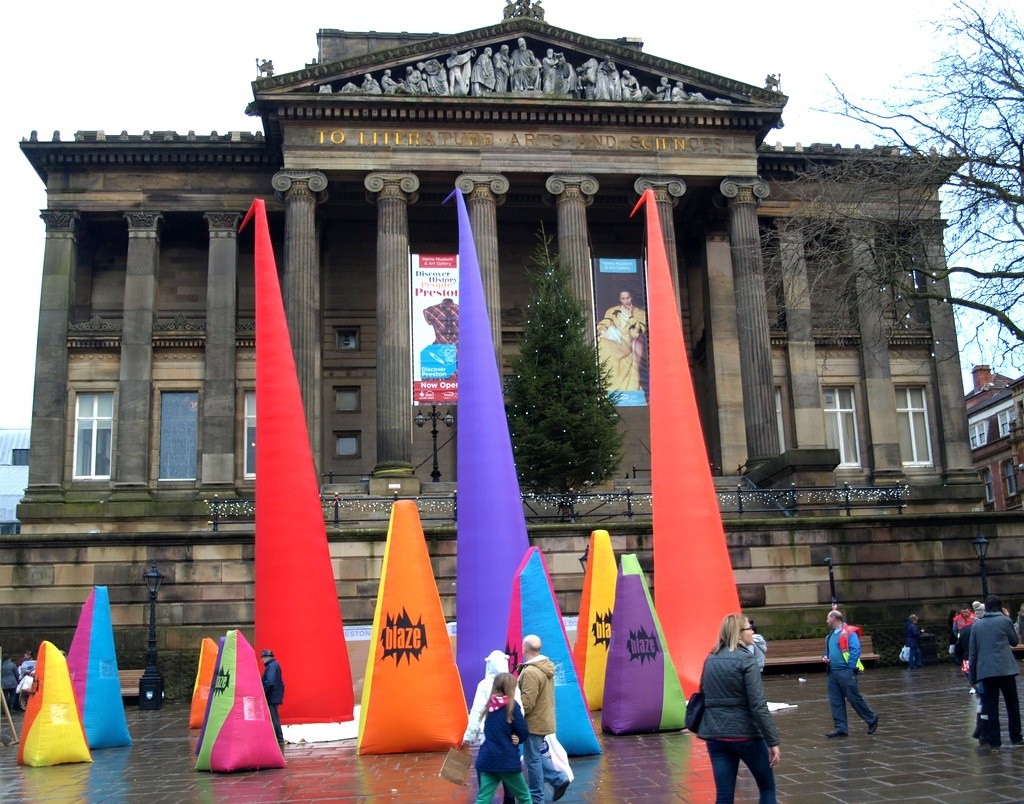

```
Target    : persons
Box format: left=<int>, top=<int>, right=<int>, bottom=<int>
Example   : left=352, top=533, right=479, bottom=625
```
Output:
left=596, top=287, right=646, bottom=398
left=969, top=595, right=1024, bottom=754
left=2, top=650, right=34, bottom=715
left=904, top=613, right=925, bottom=670
left=747, top=620, right=767, bottom=674
left=948, top=601, right=1024, bottom=747
left=260, top=650, right=284, bottom=746
left=463, top=634, right=575, bottom=804
left=822, top=610, right=879, bottom=737
left=361, top=37, right=688, bottom=101
left=696, top=614, right=780, bottom=804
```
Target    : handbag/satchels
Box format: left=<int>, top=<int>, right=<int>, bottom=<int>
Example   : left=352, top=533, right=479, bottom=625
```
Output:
left=949, top=644, right=954, bottom=654
left=544, top=732, right=574, bottom=782
left=903, top=646, right=916, bottom=662
left=15, top=675, right=29, bottom=693
left=21, top=676, right=34, bottom=693
left=962, top=660, right=970, bottom=673
left=439, top=744, right=472, bottom=785
left=685, top=693, right=706, bottom=733
left=899, top=645, right=906, bottom=661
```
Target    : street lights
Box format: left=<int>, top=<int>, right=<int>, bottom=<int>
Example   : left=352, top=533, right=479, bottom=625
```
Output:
left=972, top=533, right=990, bottom=601
left=414, top=405, right=454, bottom=482
left=139, top=561, right=165, bottom=711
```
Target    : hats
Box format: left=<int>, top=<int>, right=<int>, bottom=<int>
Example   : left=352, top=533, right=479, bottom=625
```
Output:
left=985, top=595, right=1002, bottom=612
left=909, top=615, right=918, bottom=620
left=972, top=601, right=985, bottom=609
left=60, top=650, right=65, bottom=654
left=26, top=651, right=33, bottom=656
left=261, top=650, right=273, bottom=656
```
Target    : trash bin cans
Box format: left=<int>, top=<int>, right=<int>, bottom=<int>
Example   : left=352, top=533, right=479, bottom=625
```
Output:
left=920, top=633, right=938, bottom=666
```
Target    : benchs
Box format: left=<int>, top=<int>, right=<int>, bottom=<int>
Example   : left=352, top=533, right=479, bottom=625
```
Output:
left=760, top=634, right=879, bottom=670
left=118, top=669, right=147, bottom=703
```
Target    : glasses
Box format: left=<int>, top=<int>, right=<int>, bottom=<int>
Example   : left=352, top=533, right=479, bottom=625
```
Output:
left=741, top=625, right=752, bottom=630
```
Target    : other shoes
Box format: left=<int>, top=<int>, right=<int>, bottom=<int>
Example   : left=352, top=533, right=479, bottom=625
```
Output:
left=1013, top=739, right=1024, bottom=746
left=992, top=745, right=1001, bottom=752
left=825, top=729, right=849, bottom=737
left=867, top=716, right=879, bottom=734
left=553, top=780, right=570, bottom=801
left=20, top=706, right=25, bottom=711
left=10, top=709, right=17, bottom=713
left=979, top=736, right=987, bottom=745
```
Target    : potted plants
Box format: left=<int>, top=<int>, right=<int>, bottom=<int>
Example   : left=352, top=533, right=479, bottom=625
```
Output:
left=368, top=460, right=421, bottom=496
